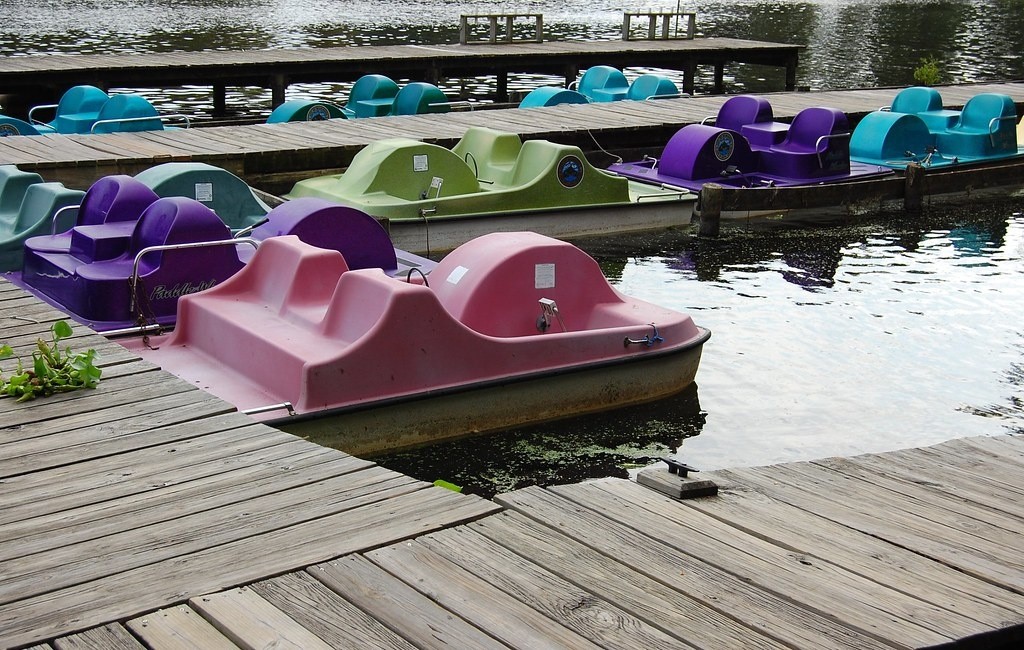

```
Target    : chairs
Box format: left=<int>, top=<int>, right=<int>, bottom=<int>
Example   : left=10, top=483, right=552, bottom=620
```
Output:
left=175, top=234, right=435, bottom=408
left=328, top=74, right=450, bottom=121
left=700, top=96, right=851, bottom=180
left=878, top=87, right=1018, bottom=158
left=568, top=65, right=680, bottom=103
left=27, top=85, right=164, bottom=134
left=24, top=174, right=231, bottom=322
left=0, top=165, right=88, bottom=271
left=446, top=125, right=588, bottom=210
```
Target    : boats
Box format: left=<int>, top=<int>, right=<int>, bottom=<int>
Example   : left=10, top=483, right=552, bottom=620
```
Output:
left=0, top=160, right=292, bottom=290
left=279, top=126, right=703, bottom=255
left=265, top=74, right=483, bottom=138
left=0, top=173, right=442, bottom=331
left=515, top=64, right=689, bottom=114
left=111, top=230, right=713, bottom=460
left=604, top=94, right=897, bottom=220
left=849, top=83, right=1024, bottom=195
left=0, top=84, right=190, bottom=149
